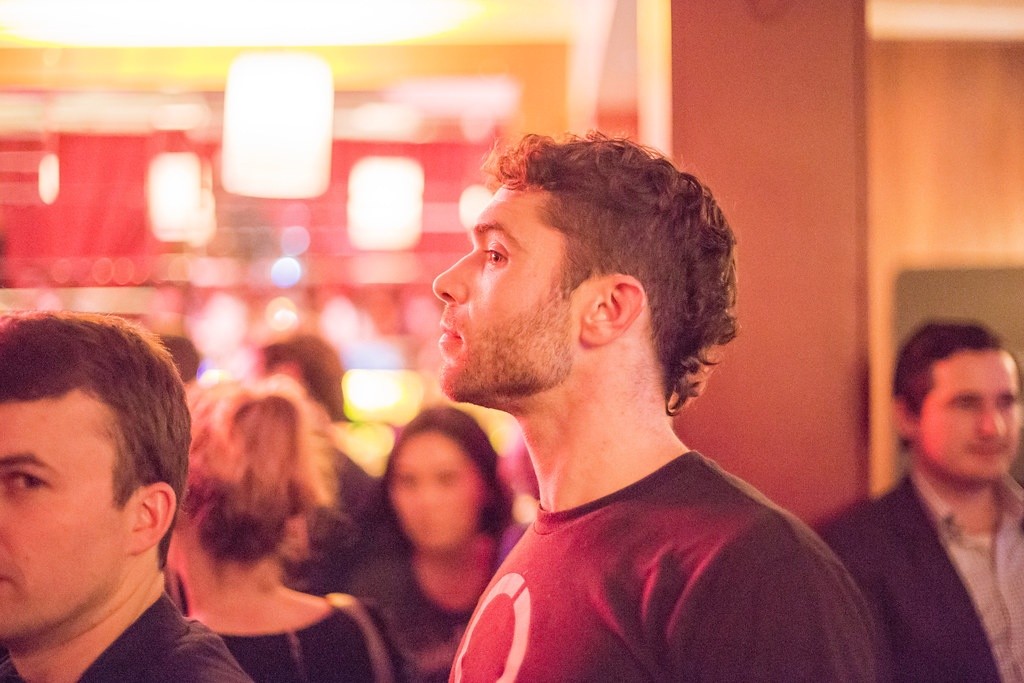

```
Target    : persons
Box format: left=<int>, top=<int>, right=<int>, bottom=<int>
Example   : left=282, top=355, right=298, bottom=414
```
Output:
left=163, top=334, right=544, bottom=683
left=812, top=322, right=1024, bottom=683
left=431, top=132, right=879, bottom=683
left=0, top=309, right=252, bottom=683
